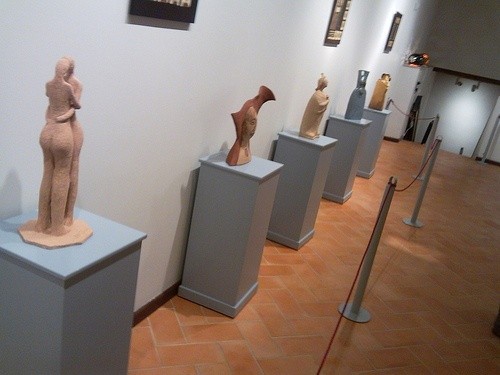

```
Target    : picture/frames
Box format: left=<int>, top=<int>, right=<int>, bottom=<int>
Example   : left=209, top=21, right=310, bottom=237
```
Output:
left=128, top=0, right=199, bottom=23
left=384, top=12, right=403, bottom=51
left=325, top=0, right=352, bottom=45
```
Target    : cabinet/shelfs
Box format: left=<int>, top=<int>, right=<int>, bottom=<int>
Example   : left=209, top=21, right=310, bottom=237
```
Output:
left=383, top=62, right=429, bottom=143
